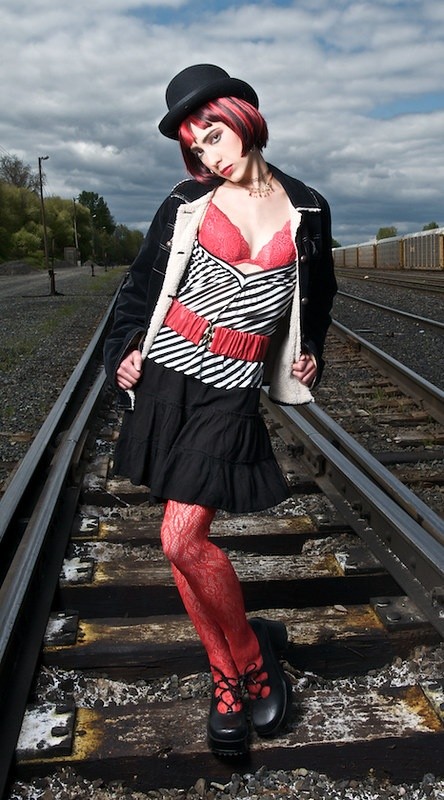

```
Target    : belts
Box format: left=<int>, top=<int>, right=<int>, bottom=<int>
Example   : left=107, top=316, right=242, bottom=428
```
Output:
left=164, top=299, right=270, bottom=362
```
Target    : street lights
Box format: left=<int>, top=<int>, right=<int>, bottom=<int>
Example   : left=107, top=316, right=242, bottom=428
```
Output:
left=102, top=226, right=108, bottom=272
left=37, top=156, right=50, bottom=271
left=91, top=214, right=97, bottom=277
left=72, top=196, right=80, bottom=249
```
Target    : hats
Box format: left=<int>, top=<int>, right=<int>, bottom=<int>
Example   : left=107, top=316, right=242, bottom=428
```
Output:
left=157, top=63, right=259, bottom=140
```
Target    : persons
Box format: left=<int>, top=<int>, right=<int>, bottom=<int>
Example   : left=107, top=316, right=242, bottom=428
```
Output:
left=102, top=62, right=338, bottom=755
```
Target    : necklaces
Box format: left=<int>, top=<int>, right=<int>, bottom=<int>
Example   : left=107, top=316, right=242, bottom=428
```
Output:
left=229, top=170, right=274, bottom=198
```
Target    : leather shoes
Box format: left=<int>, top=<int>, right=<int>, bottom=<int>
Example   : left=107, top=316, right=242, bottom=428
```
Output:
left=206, top=664, right=251, bottom=761
left=238, top=616, right=292, bottom=738
